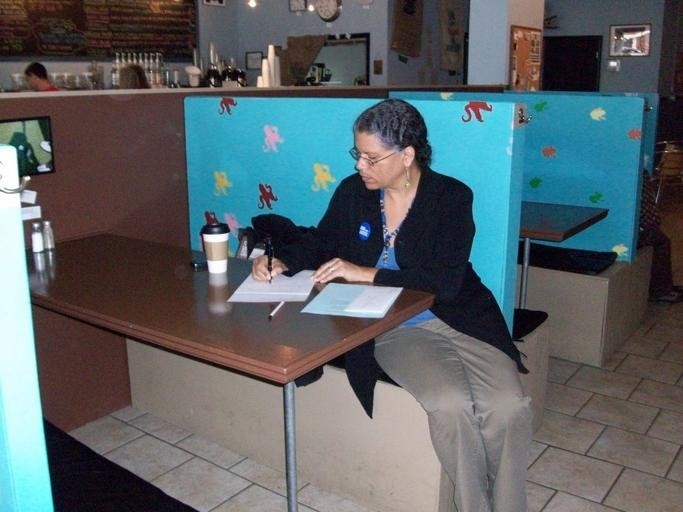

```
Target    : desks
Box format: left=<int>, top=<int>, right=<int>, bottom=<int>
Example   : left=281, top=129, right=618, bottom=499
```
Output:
left=26, top=230, right=437, bottom=511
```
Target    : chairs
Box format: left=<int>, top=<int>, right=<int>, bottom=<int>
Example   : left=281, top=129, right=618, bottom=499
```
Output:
left=655, top=139, right=683, bottom=204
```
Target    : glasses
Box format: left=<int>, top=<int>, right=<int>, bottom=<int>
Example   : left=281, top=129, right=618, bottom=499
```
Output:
left=348, top=149, right=399, bottom=166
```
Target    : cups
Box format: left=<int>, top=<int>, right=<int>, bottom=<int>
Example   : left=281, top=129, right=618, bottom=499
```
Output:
left=200, top=223, right=230, bottom=273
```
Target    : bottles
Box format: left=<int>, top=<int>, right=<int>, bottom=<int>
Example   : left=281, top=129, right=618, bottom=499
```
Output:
left=30, top=221, right=55, bottom=253
left=110, top=53, right=178, bottom=87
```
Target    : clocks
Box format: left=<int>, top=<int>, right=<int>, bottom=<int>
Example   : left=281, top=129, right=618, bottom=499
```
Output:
left=315, top=0, right=342, bottom=22
left=289, top=0, right=308, bottom=12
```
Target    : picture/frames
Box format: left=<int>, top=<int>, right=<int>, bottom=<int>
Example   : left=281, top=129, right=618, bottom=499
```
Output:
left=609, top=24, right=652, bottom=57
left=246, top=52, right=262, bottom=70
left=203, top=0, right=225, bottom=8
left=510, top=25, right=542, bottom=91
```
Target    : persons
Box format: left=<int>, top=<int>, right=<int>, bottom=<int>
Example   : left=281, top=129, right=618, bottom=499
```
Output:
left=117, top=64, right=149, bottom=89
left=636, top=169, right=683, bottom=303
left=22, top=62, right=60, bottom=91
left=251, top=99, right=533, bottom=512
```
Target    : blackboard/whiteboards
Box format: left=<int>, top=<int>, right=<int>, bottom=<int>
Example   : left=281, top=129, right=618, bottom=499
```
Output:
left=0, top=0, right=200, bottom=62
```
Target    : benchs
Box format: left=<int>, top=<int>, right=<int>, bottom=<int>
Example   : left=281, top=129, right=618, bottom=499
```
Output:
left=123, top=95, right=555, bottom=511
left=389, top=90, right=661, bottom=369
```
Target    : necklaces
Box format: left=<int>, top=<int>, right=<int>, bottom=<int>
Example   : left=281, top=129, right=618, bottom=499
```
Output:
left=379, top=189, right=413, bottom=266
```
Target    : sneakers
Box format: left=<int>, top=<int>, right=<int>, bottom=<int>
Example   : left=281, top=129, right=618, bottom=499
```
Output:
left=649, top=286, right=683, bottom=303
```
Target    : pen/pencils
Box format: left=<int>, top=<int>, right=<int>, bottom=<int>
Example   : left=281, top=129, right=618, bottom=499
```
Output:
left=267, top=240, right=273, bottom=284
left=268, top=301, right=284, bottom=319
